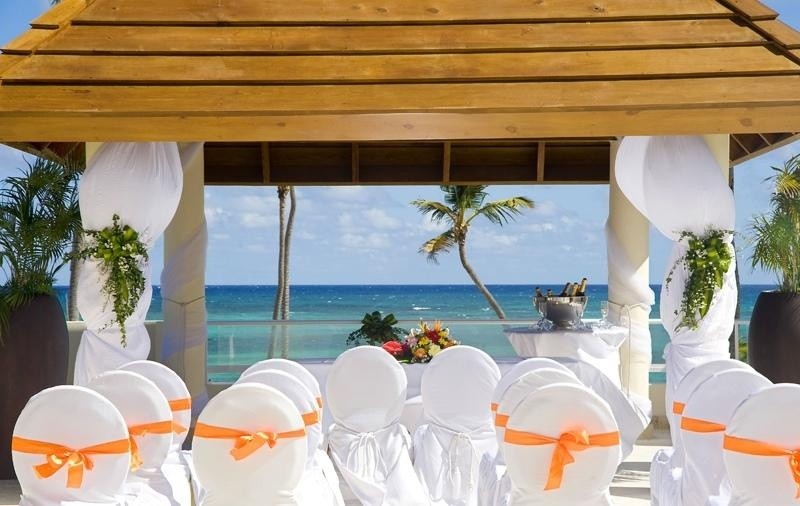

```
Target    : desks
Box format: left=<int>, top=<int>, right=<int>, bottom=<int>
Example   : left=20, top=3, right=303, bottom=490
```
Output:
left=503, top=321, right=630, bottom=392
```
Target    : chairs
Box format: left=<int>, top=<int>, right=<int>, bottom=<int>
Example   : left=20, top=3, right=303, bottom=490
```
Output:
left=325, top=347, right=416, bottom=506
left=646, top=360, right=753, bottom=503
left=240, top=357, right=323, bottom=411
left=663, top=369, right=771, bottom=506
left=491, top=358, right=571, bottom=426
left=416, top=344, right=502, bottom=506
left=501, top=383, right=622, bottom=506
left=236, top=369, right=338, bottom=505
left=496, top=369, right=585, bottom=445
left=124, top=359, right=198, bottom=489
left=13, top=384, right=144, bottom=506
left=724, top=381, right=800, bottom=506
left=192, top=383, right=320, bottom=506
left=89, top=372, right=179, bottom=503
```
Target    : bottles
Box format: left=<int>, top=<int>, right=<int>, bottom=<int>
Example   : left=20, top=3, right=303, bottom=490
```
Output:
left=536, top=276, right=588, bottom=296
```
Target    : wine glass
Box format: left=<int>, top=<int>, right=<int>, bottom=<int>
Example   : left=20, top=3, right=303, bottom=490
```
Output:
left=574, top=301, right=585, bottom=327
left=600, top=300, right=610, bottom=325
left=535, top=302, right=549, bottom=329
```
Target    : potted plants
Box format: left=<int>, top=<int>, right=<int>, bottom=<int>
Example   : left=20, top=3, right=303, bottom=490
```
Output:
left=736, top=153, right=800, bottom=383
left=0, top=144, right=97, bottom=481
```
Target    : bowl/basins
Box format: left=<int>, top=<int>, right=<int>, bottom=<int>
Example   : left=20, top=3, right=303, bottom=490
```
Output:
left=529, top=299, right=587, bottom=325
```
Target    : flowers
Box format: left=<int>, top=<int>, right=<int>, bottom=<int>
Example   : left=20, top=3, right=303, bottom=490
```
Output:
left=72, top=213, right=150, bottom=349
left=402, top=317, right=459, bottom=363
left=664, top=224, right=753, bottom=335
left=346, top=310, right=410, bottom=346
